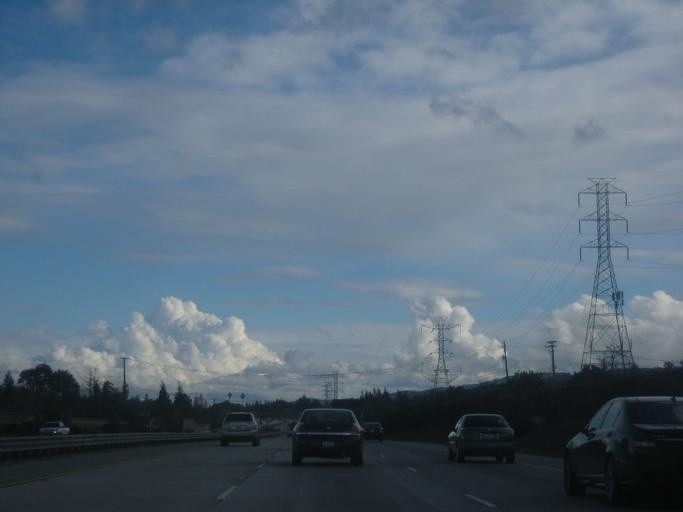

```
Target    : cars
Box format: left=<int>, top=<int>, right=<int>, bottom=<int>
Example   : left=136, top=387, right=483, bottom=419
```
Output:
left=447, top=413, right=519, bottom=468
left=38, top=420, right=71, bottom=438
left=289, top=406, right=383, bottom=467
left=562, top=394, right=682, bottom=509
left=218, top=411, right=262, bottom=448
